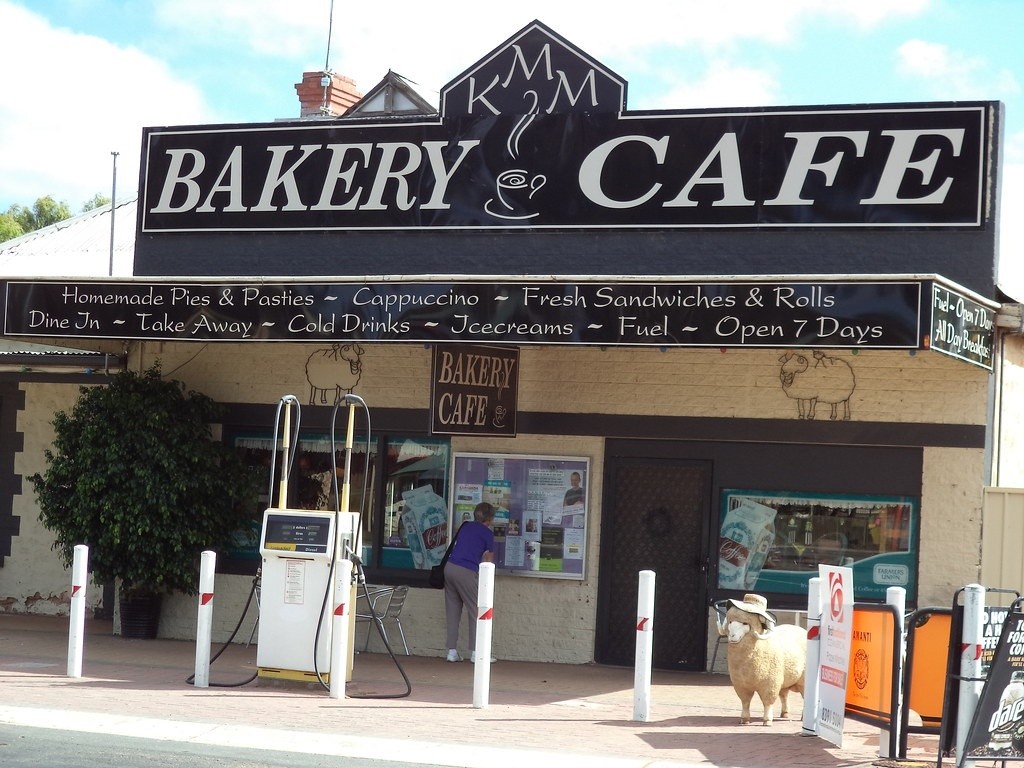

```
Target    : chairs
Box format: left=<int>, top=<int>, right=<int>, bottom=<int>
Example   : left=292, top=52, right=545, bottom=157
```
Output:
left=354, top=585, right=409, bottom=656
left=246, top=578, right=261, bottom=649
left=709, top=597, right=777, bottom=674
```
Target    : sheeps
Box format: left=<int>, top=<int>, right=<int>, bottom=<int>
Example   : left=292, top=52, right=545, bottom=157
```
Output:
left=713, top=593, right=807, bottom=726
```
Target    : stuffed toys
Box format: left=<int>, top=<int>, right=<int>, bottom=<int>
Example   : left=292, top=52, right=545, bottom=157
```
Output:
left=716, top=607, right=808, bottom=725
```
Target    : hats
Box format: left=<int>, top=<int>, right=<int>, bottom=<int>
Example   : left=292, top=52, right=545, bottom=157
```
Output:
left=726, top=594, right=777, bottom=623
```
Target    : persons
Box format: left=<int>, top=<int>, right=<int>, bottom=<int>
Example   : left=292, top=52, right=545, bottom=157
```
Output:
left=563, top=471, right=585, bottom=507
left=443, top=502, right=498, bottom=665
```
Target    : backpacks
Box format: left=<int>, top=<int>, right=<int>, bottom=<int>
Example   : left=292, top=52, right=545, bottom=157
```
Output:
left=430, top=565, right=444, bottom=589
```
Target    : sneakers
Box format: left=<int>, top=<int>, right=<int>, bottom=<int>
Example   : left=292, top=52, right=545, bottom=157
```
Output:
left=470, top=650, right=497, bottom=663
left=447, top=652, right=463, bottom=662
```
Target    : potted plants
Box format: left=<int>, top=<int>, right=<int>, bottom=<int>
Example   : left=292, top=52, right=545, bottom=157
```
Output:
left=24, top=356, right=267, bottom=639
